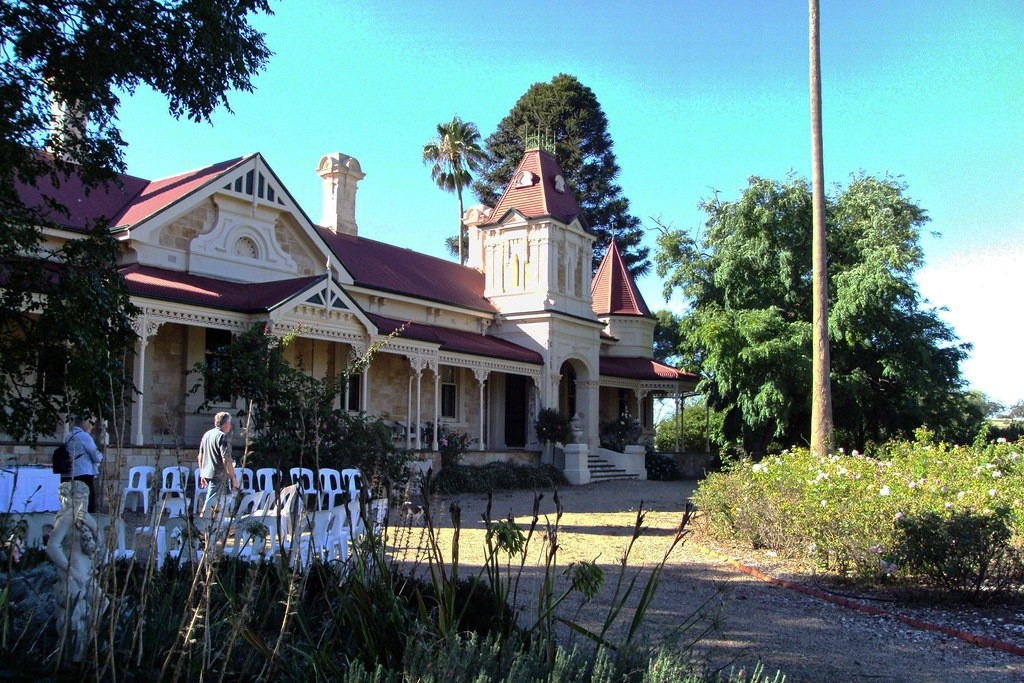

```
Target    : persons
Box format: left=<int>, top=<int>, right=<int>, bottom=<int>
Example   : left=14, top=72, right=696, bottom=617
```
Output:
left=46, top=480, right=110, bottom=658
left=59, top=412, right=104, bottom=513
left=197, top=412, right=238, bottom=541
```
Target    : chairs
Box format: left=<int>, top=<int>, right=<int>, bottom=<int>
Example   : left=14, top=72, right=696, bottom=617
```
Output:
left=98, top=464, right=390, bottom=570
left=86, top=513, right=136, bottom=580
left=10, top=512, right=59, bottom=554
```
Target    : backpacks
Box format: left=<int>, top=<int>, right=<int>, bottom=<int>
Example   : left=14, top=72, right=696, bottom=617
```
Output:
left=53, top=431, right=87, bottom=474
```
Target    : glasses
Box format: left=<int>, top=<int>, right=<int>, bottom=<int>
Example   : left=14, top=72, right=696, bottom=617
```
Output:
left=87, top=419, right=95, bottom=427
